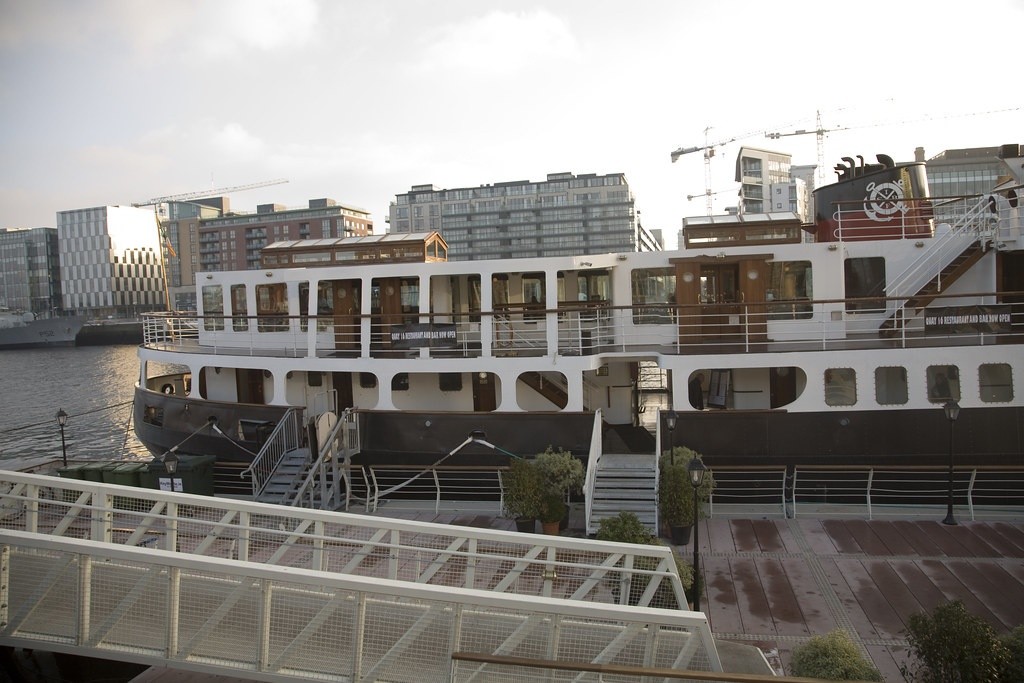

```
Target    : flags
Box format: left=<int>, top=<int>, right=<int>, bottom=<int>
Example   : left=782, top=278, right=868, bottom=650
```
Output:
left=155, top=211, right=176, bottom=257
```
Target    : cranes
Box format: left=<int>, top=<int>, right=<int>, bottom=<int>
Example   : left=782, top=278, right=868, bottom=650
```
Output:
left=764, top=109, right=850, bottom=186
left=132, top=177, right=290, bottom=284
left=669, top=125, right=767, bottom=215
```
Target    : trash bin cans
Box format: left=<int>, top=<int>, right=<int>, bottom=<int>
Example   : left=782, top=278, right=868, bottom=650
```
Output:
left=81, top=460, right=160, bottom=513
left=147, top=452, right=217, bottom=517
left=56, top=463, right=87, bottom=502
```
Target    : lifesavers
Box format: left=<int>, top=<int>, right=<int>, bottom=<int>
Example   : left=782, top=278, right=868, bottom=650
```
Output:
left=162, top=383, right=174, bottom=394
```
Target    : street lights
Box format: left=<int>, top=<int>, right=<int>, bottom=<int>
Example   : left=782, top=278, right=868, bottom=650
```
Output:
left=55, top=407, right=67, bottom=467
left=686, top=451, right=708, bottom=612
left=662, top=404, right=679, bottom=466
left=942, top=397, right=962, bottom=527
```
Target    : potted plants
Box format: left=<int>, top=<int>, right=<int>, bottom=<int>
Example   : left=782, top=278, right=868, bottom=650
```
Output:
left=663, top=488, right=699, bottom=546
left=501, top=456, right=540, bottom=533
left=593, top=510, right=658, bottom=603
left=537, top=492, right=570, bottom=534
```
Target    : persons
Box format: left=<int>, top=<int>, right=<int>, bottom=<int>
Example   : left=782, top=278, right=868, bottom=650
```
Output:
left=689, top=373, right=705, bottom=410
left=300, top=289, right=308, bottom=314
left=269, top=286, right=277, bottom=310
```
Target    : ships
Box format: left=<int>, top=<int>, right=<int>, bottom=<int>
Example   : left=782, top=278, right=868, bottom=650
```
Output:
left=132, top=154, right=1024, bottom=507
